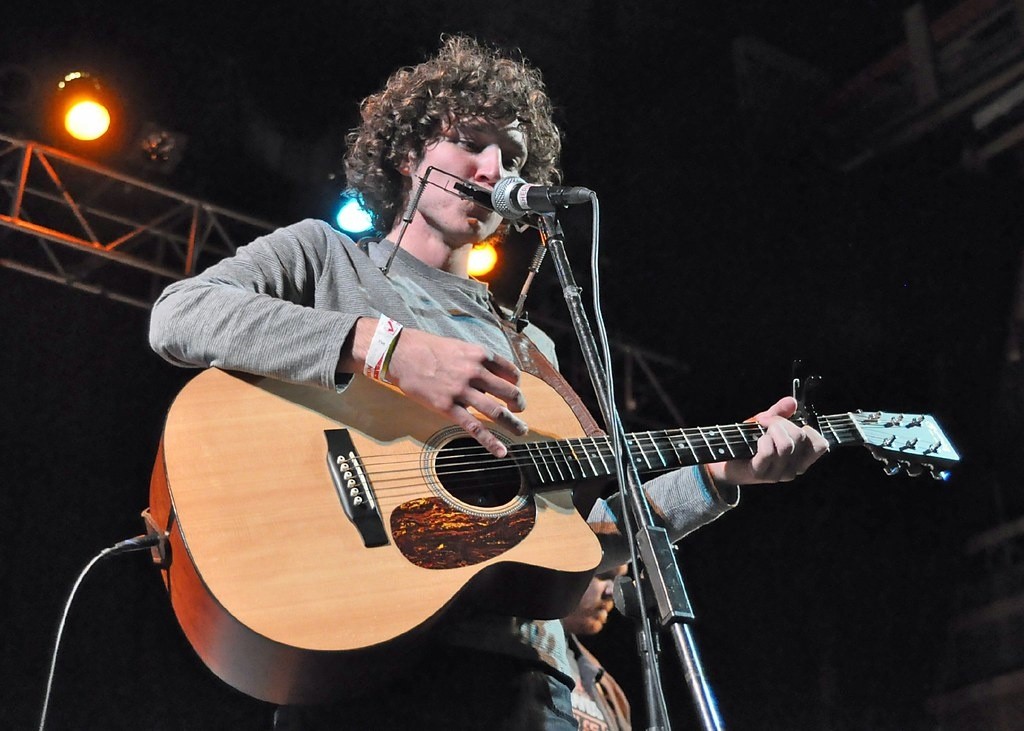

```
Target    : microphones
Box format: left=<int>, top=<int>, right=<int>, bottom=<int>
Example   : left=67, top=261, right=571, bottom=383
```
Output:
left=492, top=176, right=595, bottom=221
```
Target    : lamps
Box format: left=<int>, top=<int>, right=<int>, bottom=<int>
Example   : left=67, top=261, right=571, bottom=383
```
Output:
left=136, top=119, right=189, bottom=173
left=0, top=59, right=39, bottom=110
left=50, top=72, right=117, bottom=149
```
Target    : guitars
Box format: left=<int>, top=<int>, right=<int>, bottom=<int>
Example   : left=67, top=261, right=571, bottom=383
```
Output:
left=146, top=360, right=961, bottom=708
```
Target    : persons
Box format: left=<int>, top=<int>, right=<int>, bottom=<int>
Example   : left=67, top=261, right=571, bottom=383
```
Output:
left=148, top=36, right=831, bottom=731
left=559, top=564, right=633, bottom=731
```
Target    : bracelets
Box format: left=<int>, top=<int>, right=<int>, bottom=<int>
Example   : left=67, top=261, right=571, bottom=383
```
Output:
left=363, top=316, right=403, bottom=384
